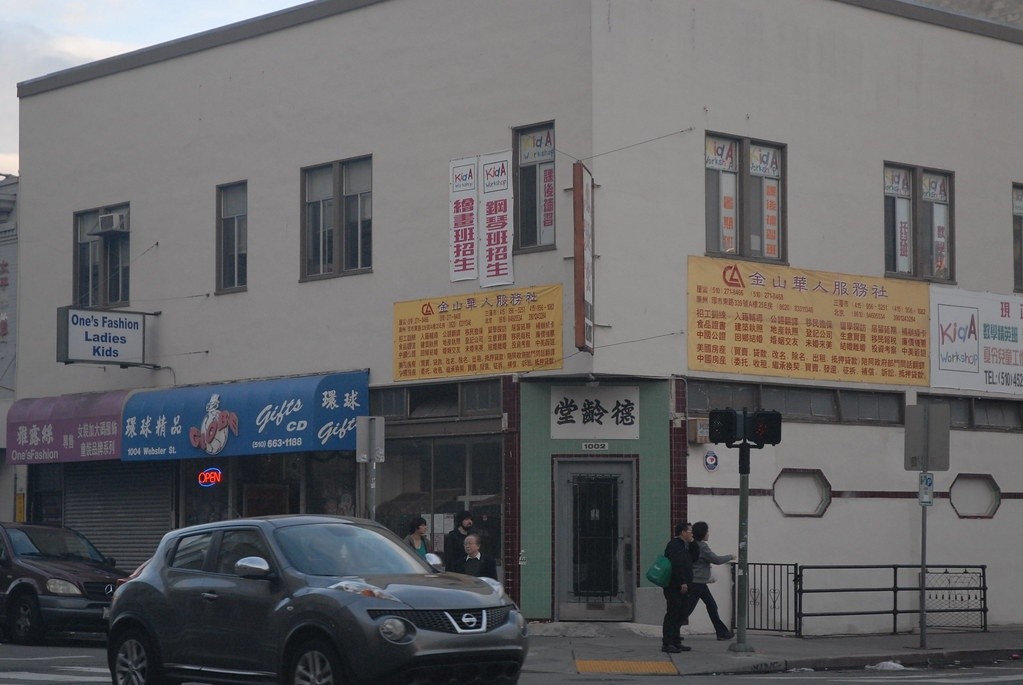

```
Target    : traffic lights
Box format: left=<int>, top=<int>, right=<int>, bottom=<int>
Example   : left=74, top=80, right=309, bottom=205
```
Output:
left=708, top=408, right=737, bottom=443
left=745, top=409, right=782, bottom=446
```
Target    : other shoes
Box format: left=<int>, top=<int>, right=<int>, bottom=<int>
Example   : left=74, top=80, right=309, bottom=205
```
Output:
left=662, top=644, right=682, bottom=653
left=674, top=643, right=691, bottom=651
left=717, top=631, right=735, bottom=641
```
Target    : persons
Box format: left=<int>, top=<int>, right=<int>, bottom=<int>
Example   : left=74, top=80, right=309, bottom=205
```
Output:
left=456, top=533, right=498, bottom=583
left=678, top=520, right=736, bottom=641
left=659, top=522, right=700, bottom=653
left=402, top=517, right=434, bottom=561
left=444, top=510, right=478, bottom=573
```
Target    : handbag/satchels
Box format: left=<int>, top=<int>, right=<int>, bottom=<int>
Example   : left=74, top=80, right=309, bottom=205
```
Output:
left=646, top=555, right=673, bottom=588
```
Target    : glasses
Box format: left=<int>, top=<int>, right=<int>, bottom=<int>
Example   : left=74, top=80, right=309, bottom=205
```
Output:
left=463, top=543, right=478, bottom=546
left=418, top=525, right=426, bottom=527
left=684, top=530, right=693, bottom=533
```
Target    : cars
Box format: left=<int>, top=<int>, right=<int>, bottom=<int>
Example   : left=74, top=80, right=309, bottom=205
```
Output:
left=106, top=515, right=527, bottom=685
left=2, top=518, right=136, bottom=642
